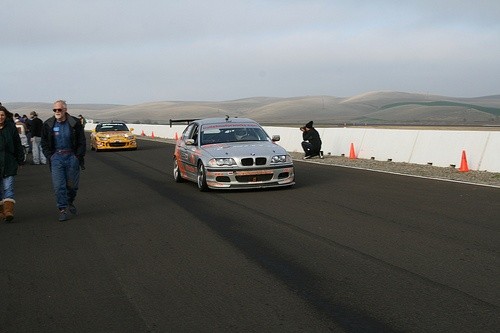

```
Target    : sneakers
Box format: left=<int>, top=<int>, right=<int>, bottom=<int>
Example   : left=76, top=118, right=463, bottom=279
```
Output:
left=57, top=204, right=76, bottom=220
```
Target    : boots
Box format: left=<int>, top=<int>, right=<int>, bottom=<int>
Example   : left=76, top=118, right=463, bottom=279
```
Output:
left=0, top=198, right=16, bottom=223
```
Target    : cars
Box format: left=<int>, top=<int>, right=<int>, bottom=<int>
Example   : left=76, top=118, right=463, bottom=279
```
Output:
left=89, top=122, right=137, bottom=152
left=172, top=117, right=296, bottom=192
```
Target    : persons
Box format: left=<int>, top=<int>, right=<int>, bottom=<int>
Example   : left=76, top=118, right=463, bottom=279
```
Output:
left=78, top=113, right=87, bottom=128
left=28, top=111, right=48, bottom=166
left=0, top=105, right=25, bottom=222
left=10, top=110, right=31, bottom=151
left=13, top=116, right=30, bottom=165
left=41, top=100, right=87, bottom=221
left=300, top=121, right=324, bottom=159
left=226, top=128, right=250, bottom=142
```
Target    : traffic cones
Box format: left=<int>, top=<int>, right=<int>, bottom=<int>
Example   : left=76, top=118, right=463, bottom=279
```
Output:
left=141, top=130, right=144, bottom=137
left=176, top=132, right=178, bottom=140
left=150, top=131, right=155, bottom=139
left=458, top=150, right=469, bottom=172
left=349, top=143, right=356, bottom=162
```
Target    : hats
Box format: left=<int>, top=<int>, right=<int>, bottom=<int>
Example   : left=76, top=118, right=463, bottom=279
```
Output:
left=305, top=120, right=313, bottom=129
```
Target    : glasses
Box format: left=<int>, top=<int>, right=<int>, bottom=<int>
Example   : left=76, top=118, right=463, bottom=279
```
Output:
left=53, top=109, right=65, bottom=112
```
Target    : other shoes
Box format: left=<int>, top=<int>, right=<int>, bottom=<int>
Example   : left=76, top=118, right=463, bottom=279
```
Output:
left=304, top=153, right=311, bottom=159
left=319, top=151, right=324, bottom=160
